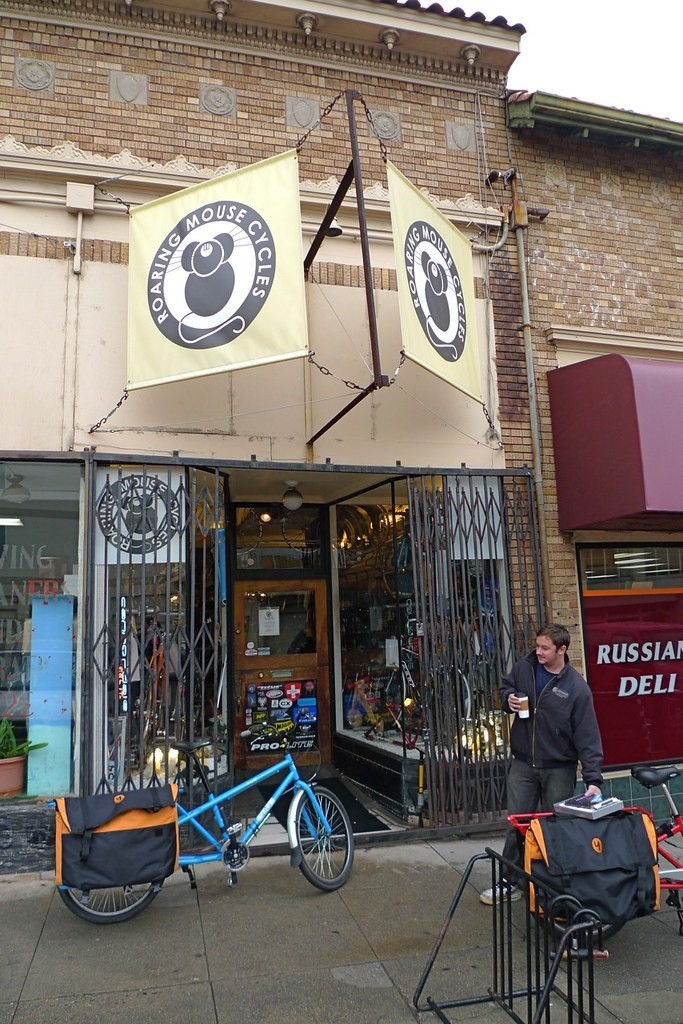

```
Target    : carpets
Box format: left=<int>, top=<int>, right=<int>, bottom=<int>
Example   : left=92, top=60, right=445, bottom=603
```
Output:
left=257, top=777, right=390, bottom=839
left=233, top=765, right=377, bottom=820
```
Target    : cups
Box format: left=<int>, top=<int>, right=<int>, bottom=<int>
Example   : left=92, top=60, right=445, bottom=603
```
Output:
left=515, top=692, right=530, bottom=719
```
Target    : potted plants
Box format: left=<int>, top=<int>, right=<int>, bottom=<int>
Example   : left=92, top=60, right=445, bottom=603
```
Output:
left=0, top=717, right=48, bottom=799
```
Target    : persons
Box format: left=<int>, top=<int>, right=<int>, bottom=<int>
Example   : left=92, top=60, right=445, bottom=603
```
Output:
left=479, top=624, right=603, bottom=905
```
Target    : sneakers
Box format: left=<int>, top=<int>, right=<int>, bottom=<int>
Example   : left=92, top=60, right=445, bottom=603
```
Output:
left=480, top=877, right=522, bottom=904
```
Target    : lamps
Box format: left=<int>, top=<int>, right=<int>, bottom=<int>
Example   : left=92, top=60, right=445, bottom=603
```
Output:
left=282, top=480, right=303, bottom=511
left=208, top=0, right=233, bottom=20
left=460, top=44, right=481, bottom=66
left=379, top=29, right=401, bottom=50
left=297, top=12, right=319, bottom=35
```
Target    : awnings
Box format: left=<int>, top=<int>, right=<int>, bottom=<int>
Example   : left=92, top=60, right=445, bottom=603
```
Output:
left=546, top=352, right=682, bottom=531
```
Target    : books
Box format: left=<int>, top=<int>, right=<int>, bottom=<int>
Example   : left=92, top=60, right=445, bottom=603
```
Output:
left=553, top=793, right=624, bottom=820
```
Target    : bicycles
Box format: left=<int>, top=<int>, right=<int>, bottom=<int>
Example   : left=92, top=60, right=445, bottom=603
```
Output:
left=381, top=640, right=471, bottom=752
left=46, top=706, right=355, bottom=924
left=504, top=755, right=683, bottom=946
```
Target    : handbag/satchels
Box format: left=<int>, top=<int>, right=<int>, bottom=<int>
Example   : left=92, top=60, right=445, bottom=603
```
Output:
left=525, top=809, right=663, bottom=926
left=55, top=783, right=179, bottom=889
